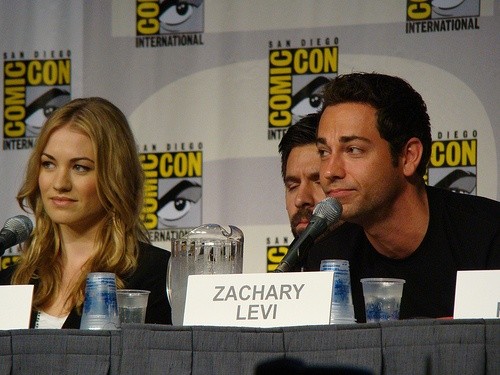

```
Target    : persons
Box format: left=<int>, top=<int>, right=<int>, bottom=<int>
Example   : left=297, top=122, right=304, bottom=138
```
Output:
left=0, top=98, right=172, bottom=330
left=279, top=112, right=326, bottom=233
left=299, top=73, right=500, bottom=316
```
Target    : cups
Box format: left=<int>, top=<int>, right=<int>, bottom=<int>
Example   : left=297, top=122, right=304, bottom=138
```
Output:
left=360, top=278, right=405, bottom=323
left=117, top=289, right=151, bottom=325
left=80, top=273, right=121, bottom=330
left=319, top=259, right=357, bottom=324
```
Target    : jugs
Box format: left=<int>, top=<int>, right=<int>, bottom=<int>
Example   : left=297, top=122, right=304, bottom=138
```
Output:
left=166, top=224, right=244, bottom=326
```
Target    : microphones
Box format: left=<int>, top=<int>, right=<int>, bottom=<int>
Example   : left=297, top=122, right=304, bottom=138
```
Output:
left=0, top=215, right=33, bottom=254
left=276, top=197, right=343, bottom=273
